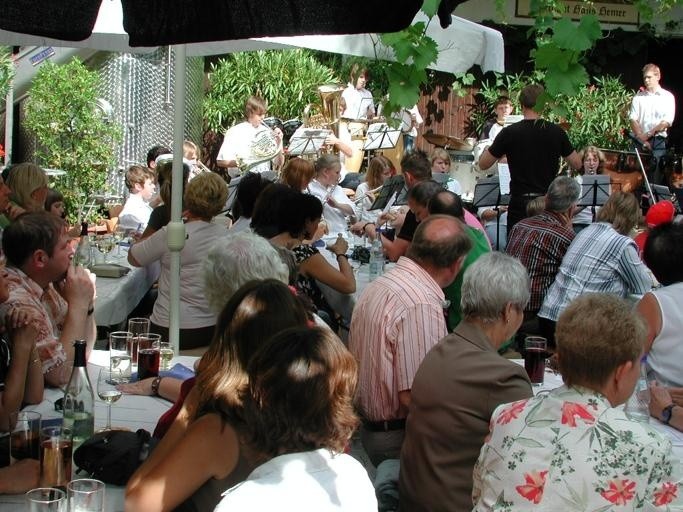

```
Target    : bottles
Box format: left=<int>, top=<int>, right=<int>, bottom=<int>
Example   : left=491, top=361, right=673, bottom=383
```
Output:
left=623, top=355, right=652, bottom=425
left=70, top=221, right=92, bottom=271
left=62, top=337, right=96, bottom=447
left=369, top=231, right=384, bottom=280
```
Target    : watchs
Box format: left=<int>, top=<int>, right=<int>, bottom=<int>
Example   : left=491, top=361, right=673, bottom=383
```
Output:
left=645, top=131, right=653, bottom=139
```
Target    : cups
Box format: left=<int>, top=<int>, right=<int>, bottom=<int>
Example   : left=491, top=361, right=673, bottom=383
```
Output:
left=105, top=314, right=175, bottom=385
left=525, top=334, right=546, bottom=387
left=7, top=410, right=73, bottom=497
left=22, top=487, right=65, bottom=512
left=66, top=477, right=105, bottom=512
left=326, top=237, right=339, bottom=247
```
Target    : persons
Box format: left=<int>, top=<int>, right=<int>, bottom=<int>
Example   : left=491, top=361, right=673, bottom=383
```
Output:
left=377, top=82, right=419, bottom=153
left=337, top=63, right=374, bottom=176
left=627, top=64, right=677, bottom=161
left=1, top=85, right=683, bottom=511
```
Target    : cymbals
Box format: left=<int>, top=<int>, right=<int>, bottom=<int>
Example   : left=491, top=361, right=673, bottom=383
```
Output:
left=422, top=133, right=473, bottom=152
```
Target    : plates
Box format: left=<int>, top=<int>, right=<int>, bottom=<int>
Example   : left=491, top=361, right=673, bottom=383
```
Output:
left=325, top=235, right=377, bottom=247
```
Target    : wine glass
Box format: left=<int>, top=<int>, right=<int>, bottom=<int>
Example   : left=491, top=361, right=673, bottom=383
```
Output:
left=94, top=366, right=123, bottom=433
left=96, top=224, right=126, bottom=265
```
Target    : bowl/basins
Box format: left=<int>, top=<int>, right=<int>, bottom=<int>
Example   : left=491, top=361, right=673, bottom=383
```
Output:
left=450, top=137, right=475, bottom=152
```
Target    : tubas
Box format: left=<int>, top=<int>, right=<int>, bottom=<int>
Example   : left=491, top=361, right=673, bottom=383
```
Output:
left=304, top=83, right=343, bottom=160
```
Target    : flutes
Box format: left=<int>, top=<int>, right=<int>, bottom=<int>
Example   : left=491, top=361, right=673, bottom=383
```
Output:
left=352, top=185, right=383, bottom=204
left=322, top=177, right=341, bottom=205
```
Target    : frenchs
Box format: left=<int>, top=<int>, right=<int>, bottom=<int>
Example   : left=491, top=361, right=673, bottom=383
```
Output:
left=234, top=119, right=283, bottom=176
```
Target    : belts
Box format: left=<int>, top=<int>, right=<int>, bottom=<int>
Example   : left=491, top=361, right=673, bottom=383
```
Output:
left=361, top=418, right=405, bottom=434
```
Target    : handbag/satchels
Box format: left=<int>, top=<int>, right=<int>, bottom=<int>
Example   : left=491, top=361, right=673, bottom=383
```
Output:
left=74, top=429, right=151, bottom=486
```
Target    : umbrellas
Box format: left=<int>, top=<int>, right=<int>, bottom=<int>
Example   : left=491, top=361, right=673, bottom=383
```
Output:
left=0, top=1, right=465, bottom=49
left=0, top=0, right=505, bottom=358
